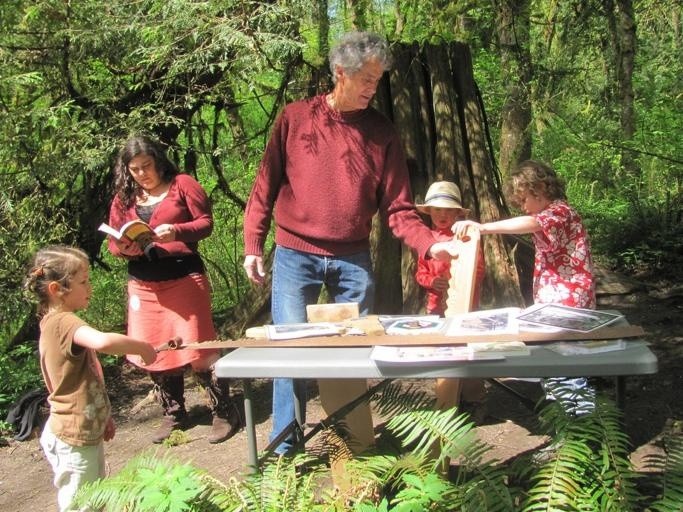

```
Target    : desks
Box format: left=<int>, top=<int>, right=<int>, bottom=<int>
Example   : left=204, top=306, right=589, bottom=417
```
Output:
left=215, top=309, right=659, bottom=474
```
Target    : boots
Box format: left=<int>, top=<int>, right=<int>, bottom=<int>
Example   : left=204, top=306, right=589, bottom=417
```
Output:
left=194, top=369, right=240, bottom=444
left=150, top=370, right=188, bottom=444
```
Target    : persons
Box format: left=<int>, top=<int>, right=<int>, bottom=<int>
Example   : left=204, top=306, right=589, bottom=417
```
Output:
left=237, top=28, right=461, bottom=480
left=20, top=243, right=158, bottom=512
left=99, top=133, right=243, bottom=447
left=413, top=179, right=488, bottom=427
left=444, top=159, right=597, bottom=463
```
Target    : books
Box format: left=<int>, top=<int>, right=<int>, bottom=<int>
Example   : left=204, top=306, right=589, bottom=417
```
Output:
left=96, top=220, right=155, bottom=247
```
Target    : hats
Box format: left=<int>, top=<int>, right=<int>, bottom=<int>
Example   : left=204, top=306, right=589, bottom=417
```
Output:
left=415, top=181, right=471, bottom=216
